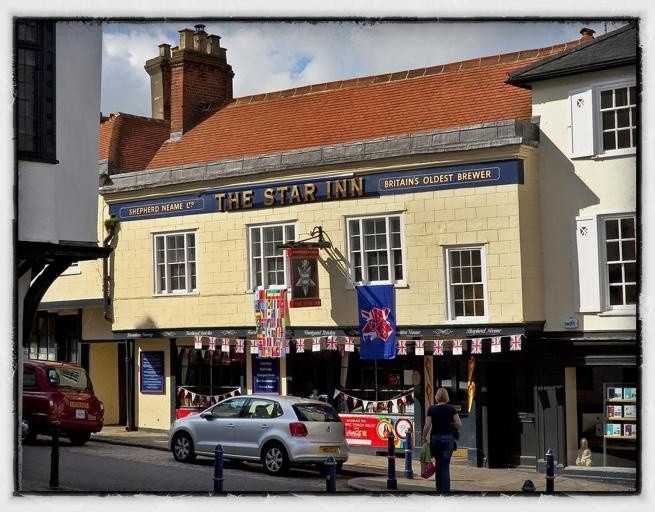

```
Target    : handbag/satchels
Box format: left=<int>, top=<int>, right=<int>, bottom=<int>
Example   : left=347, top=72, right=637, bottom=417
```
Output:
left=421, top=460, right=436, bottom=479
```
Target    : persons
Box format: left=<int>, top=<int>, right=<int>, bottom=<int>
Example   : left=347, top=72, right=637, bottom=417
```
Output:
left=422, top=388, right=462, bottom=497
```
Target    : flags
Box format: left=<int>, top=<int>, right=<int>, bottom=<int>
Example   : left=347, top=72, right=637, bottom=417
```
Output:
left=352, top=280, right=397, bottom=361
left=286, top=336, right=355, bottom=354
left=194, top=335, right=259, bottom=353
left=254, top=289, right=286, bottom=358
left=398, top=335, right=522, bottom=356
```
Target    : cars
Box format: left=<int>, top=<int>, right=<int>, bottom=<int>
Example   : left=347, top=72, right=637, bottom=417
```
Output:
left=169, top=395, right=350, bottom=476
left=24, top=360, right=106, bottom=446
